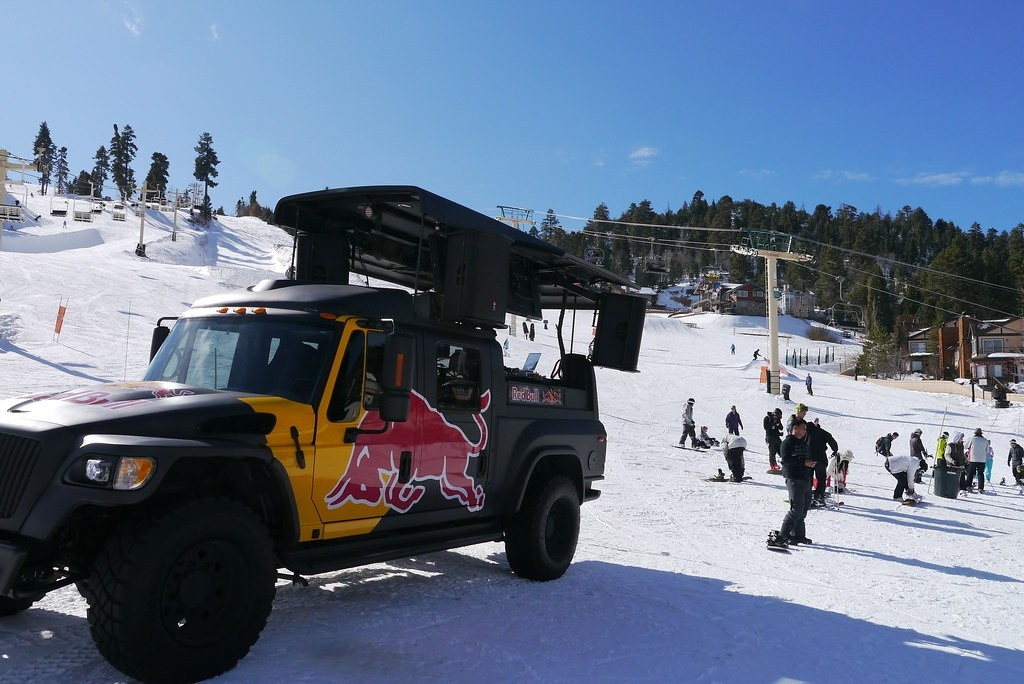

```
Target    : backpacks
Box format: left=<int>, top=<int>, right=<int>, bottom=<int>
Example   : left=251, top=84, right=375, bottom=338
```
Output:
left=873, top=437, right=890, bottom=456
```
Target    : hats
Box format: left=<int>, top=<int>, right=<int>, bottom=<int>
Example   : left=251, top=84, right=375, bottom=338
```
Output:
left=920, top=461, right=928, bottom=472
left=1010, top=439, right=1016, bottom=445
left=913, top=428, right=921, bottom=436
left=796, top=403, right=806, bottom=417
left=975, top=428, right=982, bottom=434
left=774, top=408, right=782, bottom=419
left=943, top=432, right=949, bottom=438
left=839, top=449, right=856, bottom=464
left=687, top=398, right=695, bottom=404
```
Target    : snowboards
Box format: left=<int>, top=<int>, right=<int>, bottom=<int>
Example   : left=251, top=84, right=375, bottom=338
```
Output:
left=702, top=476, right=752, bottom=482
left=902, top=498, right=915, bottom=505
left=767, top=531, right=788, bottom=550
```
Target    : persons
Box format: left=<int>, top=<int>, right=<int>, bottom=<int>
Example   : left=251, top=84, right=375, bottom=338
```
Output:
left=875, top=431, right=899, bottom=458
left=806, top=372, right=813, bottom=395
left=885, top=455, right=928, bottom=501
left=725, top=405, right=743, bottom=436
left=753, top=349, right=761, bottom=361
left=766, top=402, right=854, bottom=550
left=678, top=398, right=698, bottom=448
left=936, top=427, right=994, bottom=494
left=730, top=343, right=736, bottom=355
left=1007, top=439, right=1024, bottom=486
left=853, top=364, right=858, bottom=381
left=764, top=408, right=785, bottom=471
left=720, top=434, right=747, bottom=483
left=909, top=428, right=928, bottom=483
left=696, top=426, right=714, bottom=447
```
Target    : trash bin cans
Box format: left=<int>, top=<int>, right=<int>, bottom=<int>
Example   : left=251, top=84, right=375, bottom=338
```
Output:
left=934, top=466, right=963, bottom=499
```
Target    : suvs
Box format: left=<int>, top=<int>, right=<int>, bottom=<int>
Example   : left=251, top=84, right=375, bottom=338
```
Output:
left=0, top=182, right=647, bottom=684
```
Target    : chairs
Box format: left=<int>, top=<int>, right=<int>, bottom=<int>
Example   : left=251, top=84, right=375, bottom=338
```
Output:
left=442, top=348, right=481, bottom=410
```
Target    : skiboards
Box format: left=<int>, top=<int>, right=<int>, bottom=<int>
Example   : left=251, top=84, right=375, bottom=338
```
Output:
left=672, top=446, right=706, bottom=452
left=809, top=502, right=844, bottom=510
left=968, top=483, right=975, bottom=493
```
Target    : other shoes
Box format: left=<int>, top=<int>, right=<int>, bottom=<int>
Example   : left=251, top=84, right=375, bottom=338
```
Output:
left=795, top=536, right=812, bottom=544
left=978, top=489, right=983, bottom=494
left=679, top=442, right=685, bottom=446
left=959, top=492, right=968, bottom=496
left=910, top=493, right=922, bottom=499
left=814, top=499, right=826, bottom=507
left=967, top=486, right=972, bottom=492
left=770, top=464, right=780, bottom=473
left=893, top=497, right=904, bottom=503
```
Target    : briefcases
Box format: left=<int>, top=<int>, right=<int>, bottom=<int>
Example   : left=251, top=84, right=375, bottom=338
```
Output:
left=1017, top=465, right=1024, bottom=479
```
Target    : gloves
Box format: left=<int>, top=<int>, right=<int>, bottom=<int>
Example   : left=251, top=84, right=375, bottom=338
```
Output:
left=922, top=451, right=927, bottom=457
left=1008, top=460, right=1010, bottom=466
